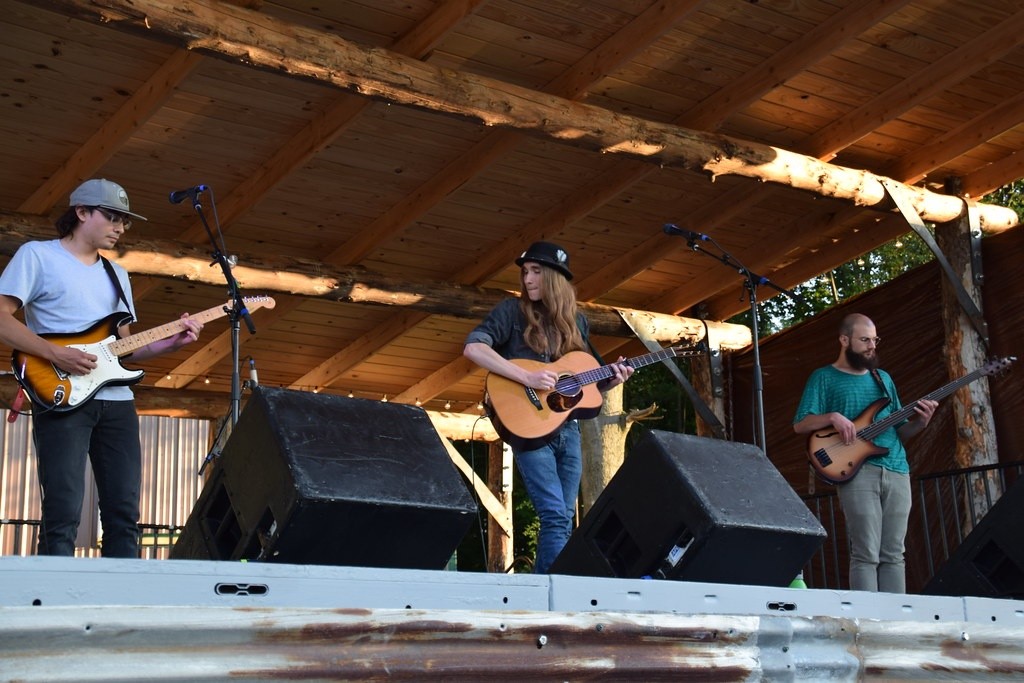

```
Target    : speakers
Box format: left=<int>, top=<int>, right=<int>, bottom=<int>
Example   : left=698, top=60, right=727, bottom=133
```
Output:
left=546, top=430, right=828, bottom=588
left=169, top=387, right=478, bottom=571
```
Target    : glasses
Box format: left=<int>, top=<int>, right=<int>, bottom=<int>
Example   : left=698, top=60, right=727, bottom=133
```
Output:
left=849, top=335, right=881, bottom=345
left=94, top=207, right=132, bottom=231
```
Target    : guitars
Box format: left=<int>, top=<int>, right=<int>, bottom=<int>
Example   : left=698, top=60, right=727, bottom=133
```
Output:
left=9, top=293, right=277, bottom=416
left=804, top=357, right=1017, bottom=486
left=481, top=340, right=709, bottom=452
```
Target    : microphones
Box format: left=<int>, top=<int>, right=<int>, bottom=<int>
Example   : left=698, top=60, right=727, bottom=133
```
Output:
left=662, top=222, right=709, bottom=241
left=169, top=184, right=207, bottom=204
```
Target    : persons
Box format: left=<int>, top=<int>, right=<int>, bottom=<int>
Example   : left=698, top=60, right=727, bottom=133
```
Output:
left=793, top=311, right=939, bottom=595
left=0, top=176, right=204, bottom=560
left=462, top=240, right=636, bottom=574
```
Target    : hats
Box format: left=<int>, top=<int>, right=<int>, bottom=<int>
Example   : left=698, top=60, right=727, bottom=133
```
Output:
left=514, top=241, right=574, bottom=281
left=69, top=178, right=148, bottom=222
